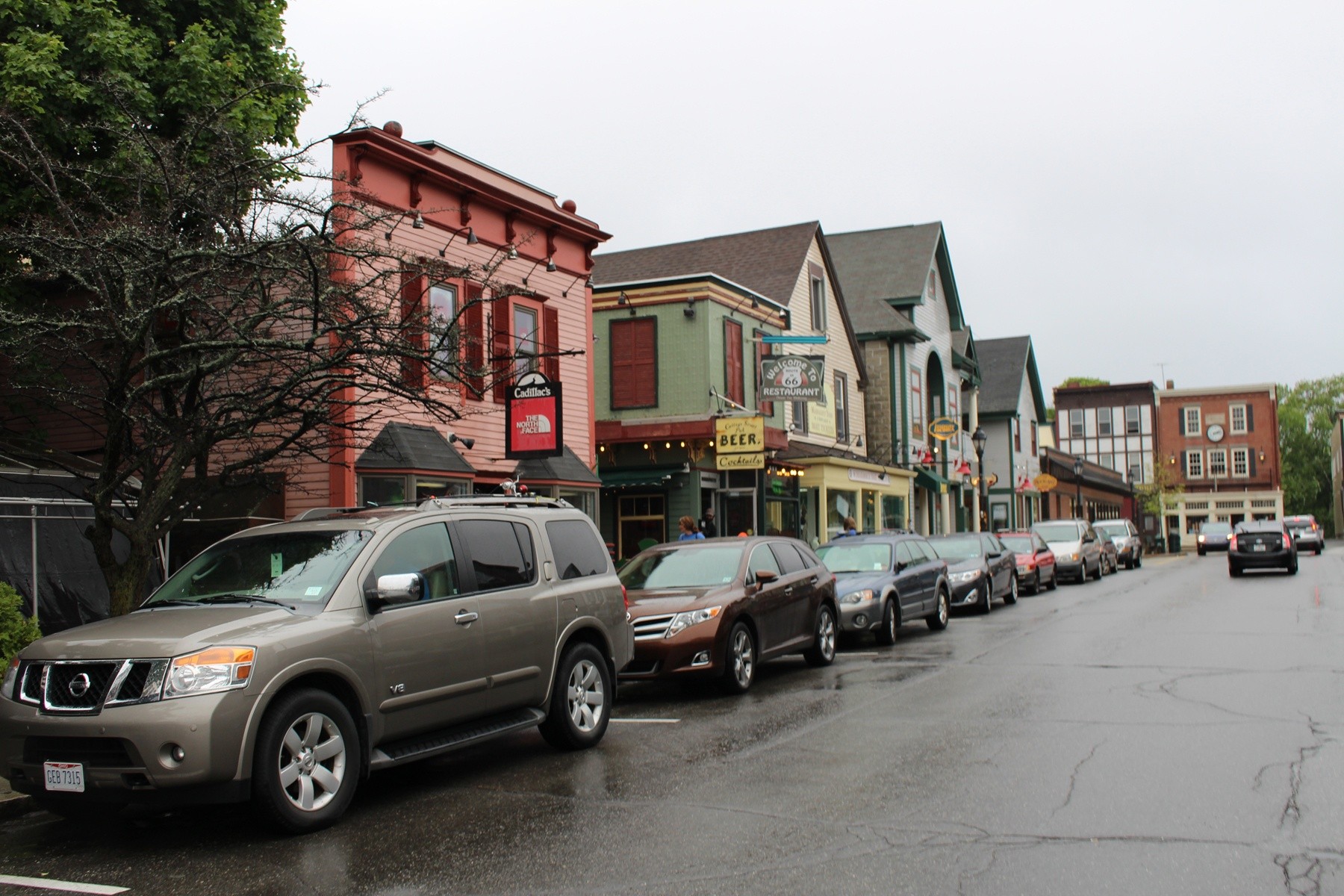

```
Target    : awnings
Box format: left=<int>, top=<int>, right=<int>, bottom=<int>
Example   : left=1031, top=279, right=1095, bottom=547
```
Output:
left=913, top=468, right=951, bottom=496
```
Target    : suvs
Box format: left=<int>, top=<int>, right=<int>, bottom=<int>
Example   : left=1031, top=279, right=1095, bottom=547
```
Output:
left=1030, top=517, right=1108, bottom=584
left=1091, top=517, right=1142, bottom=570
left=0, top=492, right=636, bottom=842
left=1282, top=514, right=1325, bottom=556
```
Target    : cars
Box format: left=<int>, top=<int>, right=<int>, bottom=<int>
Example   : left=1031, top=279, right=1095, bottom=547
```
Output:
left=1228, top=518, right=1300, bottom=578
left=1091, top=525, right=1118, bottom=576
left=925, top=531, right=1020, bottom=616
left=1196, top=521, right=1232, bottom=556
left=615, top=535, right=843, bottom=697
left=815, top=528, right=951, bottom=648
left=991, top=530, right=1058, bottom=596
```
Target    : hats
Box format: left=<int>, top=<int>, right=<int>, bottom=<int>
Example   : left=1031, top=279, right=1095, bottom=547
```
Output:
left=706, top=508, right=714, bottom=516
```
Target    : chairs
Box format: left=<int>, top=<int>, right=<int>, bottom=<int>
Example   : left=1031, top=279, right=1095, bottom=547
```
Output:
left=425, top=570, right=448, bottom=598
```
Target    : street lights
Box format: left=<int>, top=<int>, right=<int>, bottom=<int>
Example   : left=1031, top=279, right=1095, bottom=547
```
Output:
left=1127, top=469, right=1136, bottom=527
left=970, top=425, right=988, bottom=531
left=1073, top=457, right=1084, bottom=518
left=1335, top=409, right=1344, bottom=497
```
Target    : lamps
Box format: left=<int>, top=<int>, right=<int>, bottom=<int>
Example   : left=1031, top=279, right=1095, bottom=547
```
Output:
left=784, top=424, right=795, bottom=434
left=484, top=242, right=517, bottom=271
left=709, top=386, right=735, bottom=413
left=1169, top=448, right=1176, bottom=465
left=954, top=454, right=971, bottom=473
left=619, top=290, right=637, bottom=315
left=521, top=254, right=557, bottom=285
left=447, top=430, right=475, bottom=449
left=760, top=306, right=787, bottom=329
left=562, top=273, right=594, bottom=299
left=825, top=433, right=939, bottom=480
left=385, top=210, right=424, bottom=240
left=440, top=226, right=478, bottom=257
left=1258, top=445, right=1265, bottom=464
left=1019, top=459, right=1033, bottom=489
left=684, top=296, right=694, bottom=316
left=731, top=294, right=758, bottom=318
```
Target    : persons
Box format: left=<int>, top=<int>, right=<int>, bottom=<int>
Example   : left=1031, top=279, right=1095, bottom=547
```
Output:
left=842, top=518, right=856, bottom=537
left=677, top=506, right=716, bottom=543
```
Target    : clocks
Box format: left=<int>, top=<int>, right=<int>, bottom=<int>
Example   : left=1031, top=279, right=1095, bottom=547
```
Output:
left=1206, top=424, right=1224, bottom=442
